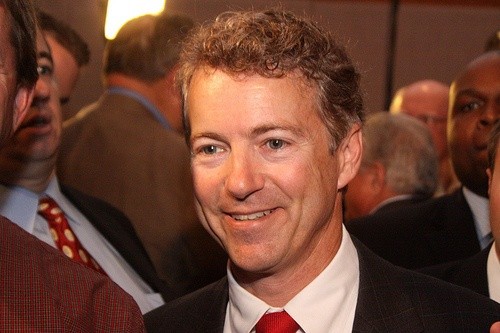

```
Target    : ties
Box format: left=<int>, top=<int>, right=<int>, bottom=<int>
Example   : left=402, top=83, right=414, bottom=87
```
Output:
left=37, top=196, right=108, bottom=277
left=255, top=310, right=301, bottom=333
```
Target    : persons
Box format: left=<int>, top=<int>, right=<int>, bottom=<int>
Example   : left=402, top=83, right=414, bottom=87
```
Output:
left=57, top=14, right=227, bottom=303
left=0, top=0, right=171, bottom=316
left=390, top=78, right=460, bottom=196
left=344, top=49, right=500, bottom=271
left=414, top=123, right=500, bottom=303
left=0, top=0, right=148, bottom=333
left=142, top=1, right=500, bottom=333
left=38, top=10, right=90, bottom=105
left=345, top=112, right=441, bottom=222
